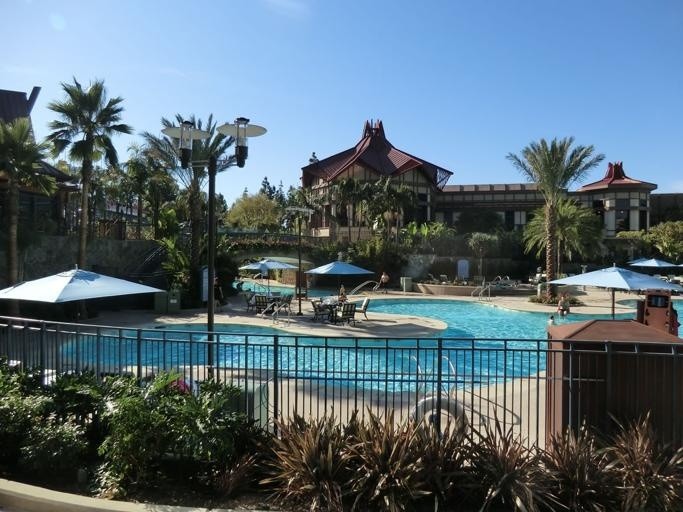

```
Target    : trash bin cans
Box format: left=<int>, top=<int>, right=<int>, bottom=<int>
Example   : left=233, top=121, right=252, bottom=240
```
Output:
left=154, top=291, right=180, bottom=314
left=399, top=276, right=412, bottom=292
left=536, top=278, right=546, bottom=298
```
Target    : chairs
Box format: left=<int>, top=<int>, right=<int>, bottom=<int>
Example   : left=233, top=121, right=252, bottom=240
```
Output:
left=243, top=291, right=294, bottom=317
left=427, top=273, right=466, bottom=285
left=311, top=296, right=370, bottom=327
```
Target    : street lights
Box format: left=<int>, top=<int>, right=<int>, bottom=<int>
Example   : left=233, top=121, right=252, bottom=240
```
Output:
left=284, top=206, right=315, bottom=316
left=161, top=114, right=266, bottom=378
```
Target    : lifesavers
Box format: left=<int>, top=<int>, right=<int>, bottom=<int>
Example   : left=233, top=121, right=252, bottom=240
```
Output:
left=408, top=395, right=469, bottom=448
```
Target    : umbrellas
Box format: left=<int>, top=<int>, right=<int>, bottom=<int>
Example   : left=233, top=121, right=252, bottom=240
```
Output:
left=630, top=259, right=683, bottom=267
left=546, top=263, right=683, bottom=319
left=238, top=257, right=300, bottom=297
left=304, top=260, right=376, bottom=292
left=626, top=257, right=649, bottom=270
left=0, top=264, right=167, bottom=323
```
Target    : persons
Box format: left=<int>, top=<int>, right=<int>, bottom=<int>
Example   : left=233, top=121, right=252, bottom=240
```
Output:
left=380, top=272, right=390, bottom=294
left=558, top=297, right=568, bottom=317
left=549, top=316, right=555, bottom=325
left=340, top=285, right=345, bottom=295
left=338, top=293, right=348, bottom=302
left=237, top=282, right=243, bottom=292
left=214, top=278, right=224, bottom=313
